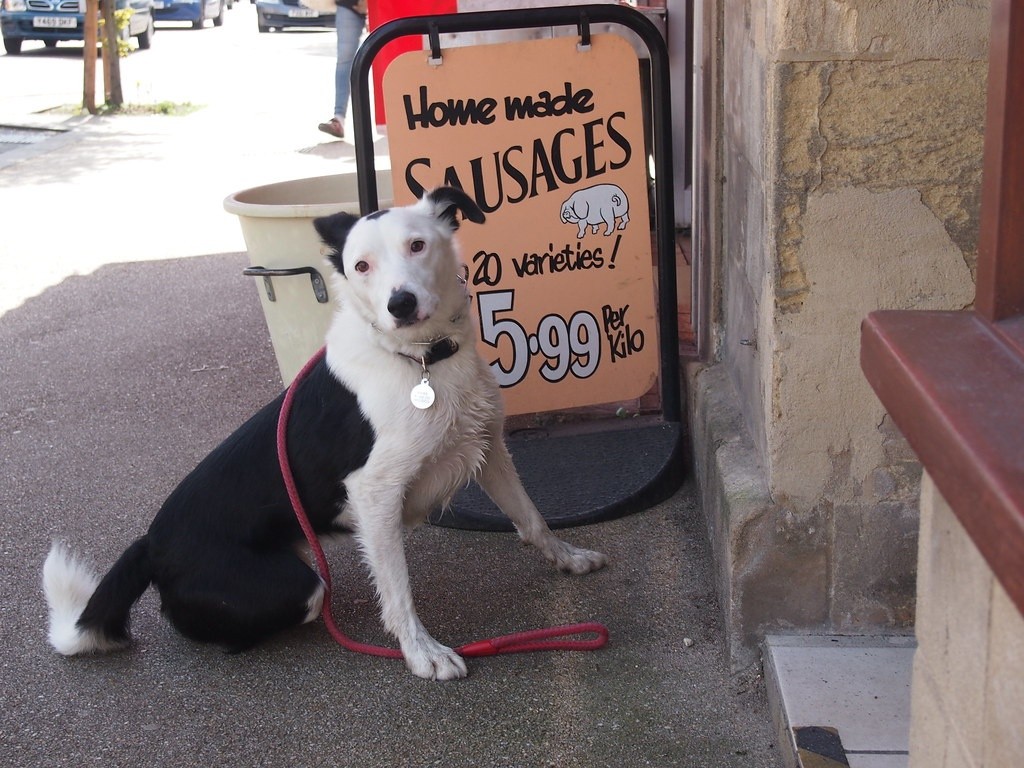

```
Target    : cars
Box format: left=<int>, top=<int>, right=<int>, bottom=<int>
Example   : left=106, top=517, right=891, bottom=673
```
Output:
left=150, top=1, right=224, bottom=32
left=0, top=0, right=155, bottom=53
left=255, top=1, right=339, bottom=32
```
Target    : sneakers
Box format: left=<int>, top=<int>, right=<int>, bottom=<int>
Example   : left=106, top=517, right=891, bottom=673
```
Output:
left=319, top=117, right=344, bottom=138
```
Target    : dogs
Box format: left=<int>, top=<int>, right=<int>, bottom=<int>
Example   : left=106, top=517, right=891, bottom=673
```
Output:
left=42, top=184, right=608, bottom=679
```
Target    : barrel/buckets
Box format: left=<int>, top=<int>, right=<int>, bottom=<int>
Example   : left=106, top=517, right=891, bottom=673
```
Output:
left=223, top=167, right=396, bottom=392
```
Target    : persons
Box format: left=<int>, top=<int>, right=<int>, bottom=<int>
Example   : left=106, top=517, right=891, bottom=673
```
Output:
left=318, top=0, right=368, bottom=140
